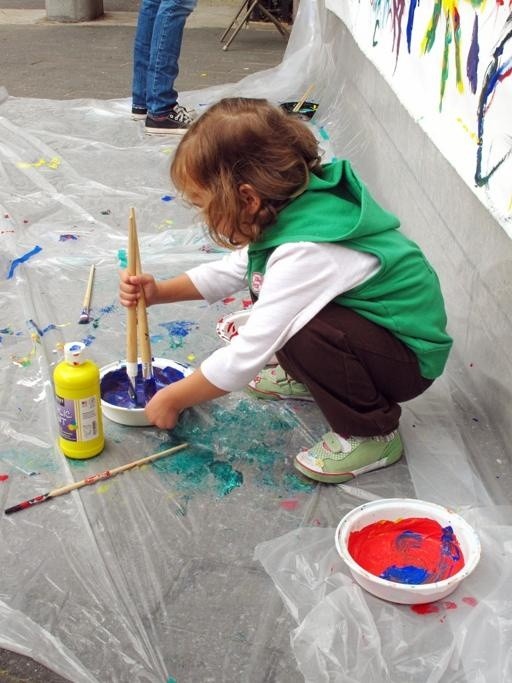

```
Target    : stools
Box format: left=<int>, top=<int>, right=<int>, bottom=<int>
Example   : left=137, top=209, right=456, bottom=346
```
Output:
left=220, top=0, right=291, bottom=51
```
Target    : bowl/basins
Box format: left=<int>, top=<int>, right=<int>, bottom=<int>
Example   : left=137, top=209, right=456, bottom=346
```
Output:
left=281, top=102, right=319, bottom=118
left=333, top=498, right=483, bottom=606
left=99, top=358, right=193, bottom=428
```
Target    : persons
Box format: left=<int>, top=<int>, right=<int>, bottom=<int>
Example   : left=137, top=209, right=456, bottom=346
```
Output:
left=130, top=0, right=200, bottom=135
left=118, top=98, right=454, bottom=483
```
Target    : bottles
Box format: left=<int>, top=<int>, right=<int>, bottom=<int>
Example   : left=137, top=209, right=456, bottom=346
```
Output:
left=53, top=341, right=105, bottom=461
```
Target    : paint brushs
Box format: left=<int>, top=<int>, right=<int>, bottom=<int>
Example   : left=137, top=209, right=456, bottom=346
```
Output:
left=292, top=84, right=313, bottom=111
left=5, top=442, right=189, bottom=514
left=130, top=207, right=157, bottom=403
left=295, top=86, right=314, bottom=112
left=79, top=264, right=95, bottom=324
left=127, top=214, right=137, bottom=401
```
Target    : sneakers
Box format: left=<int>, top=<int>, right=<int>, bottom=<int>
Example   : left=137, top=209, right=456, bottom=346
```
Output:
left=145, top=105, right=196, bottom=134
left=294, top=431, right=404, bottom=483
left=248, top=364, right=315, bottom=400
left=132, top=104, right=147, bottom=120
